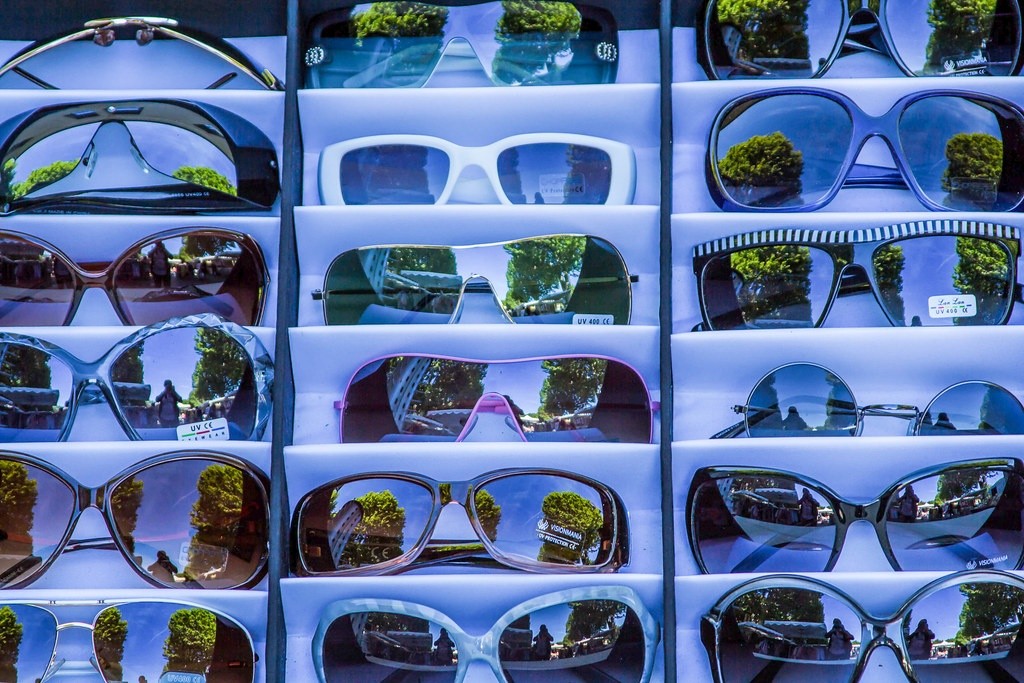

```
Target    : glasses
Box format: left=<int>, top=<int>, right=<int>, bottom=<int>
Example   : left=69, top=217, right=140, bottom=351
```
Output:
left=0, top=0, right=1024, bottom=683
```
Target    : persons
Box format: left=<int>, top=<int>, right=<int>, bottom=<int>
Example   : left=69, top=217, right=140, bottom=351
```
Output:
left=1, top=194, right=989, bottom=683
